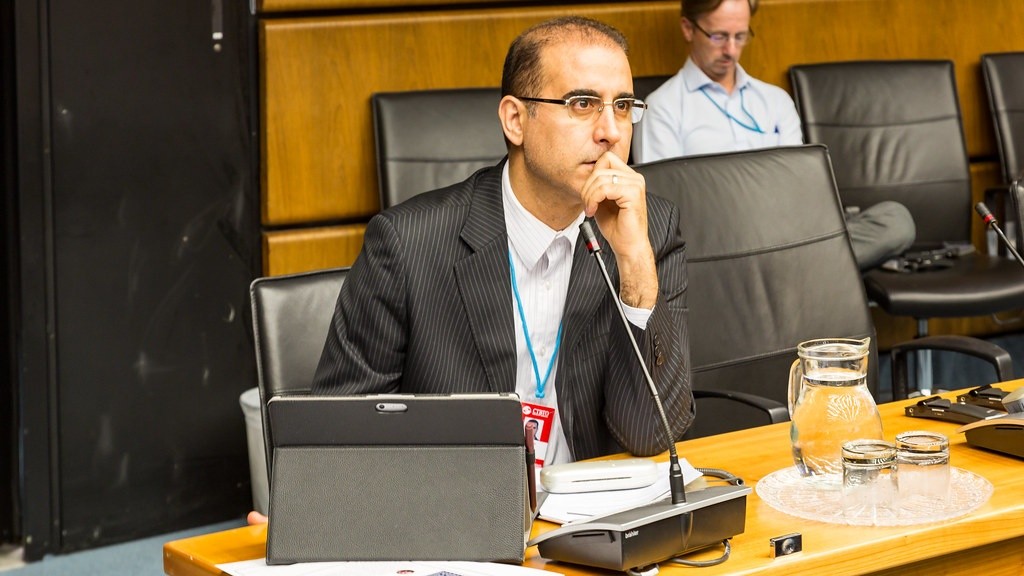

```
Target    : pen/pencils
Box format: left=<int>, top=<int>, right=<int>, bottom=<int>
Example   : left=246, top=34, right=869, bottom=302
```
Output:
left=525, top=422, right=539, bottom=516
left=774, top=124, right=779, bottom=133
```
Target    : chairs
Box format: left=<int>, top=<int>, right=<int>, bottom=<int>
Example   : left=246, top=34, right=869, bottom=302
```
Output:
left=628, top=144, right=1014, bottom=440
left=786, top=58, right=1023, bottom=398
left=371, top=87, right=509, bottom=213
left=248, top=268, right=352, bottom=396
left=979, top=51, right=1024, bottom=236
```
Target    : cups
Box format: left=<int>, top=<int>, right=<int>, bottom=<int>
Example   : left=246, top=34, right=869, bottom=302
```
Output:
left=844, top=439, right=900, bottom=509
left=895, top=430, right=949, bottom=504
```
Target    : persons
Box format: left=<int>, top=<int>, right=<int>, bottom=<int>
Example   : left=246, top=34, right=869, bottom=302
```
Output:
left=633, top=0, right=916, bottom=271
left=529, top=420, right=538, bottom=440
left=312, top=18, right=698, bottom=466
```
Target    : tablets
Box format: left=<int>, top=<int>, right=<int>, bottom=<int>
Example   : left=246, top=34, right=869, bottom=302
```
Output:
left=265, top=392, right=533, bottom=565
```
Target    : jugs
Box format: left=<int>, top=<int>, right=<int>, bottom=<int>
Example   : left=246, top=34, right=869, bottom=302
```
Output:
left=788, top=337, right=883, bottom=476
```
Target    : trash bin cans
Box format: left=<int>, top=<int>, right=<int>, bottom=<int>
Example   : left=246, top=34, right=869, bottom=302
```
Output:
left=238, top=386, right=270, bottom=520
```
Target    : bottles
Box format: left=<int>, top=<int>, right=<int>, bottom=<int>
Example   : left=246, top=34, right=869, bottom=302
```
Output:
left=846, top=205, right=860, bottom=219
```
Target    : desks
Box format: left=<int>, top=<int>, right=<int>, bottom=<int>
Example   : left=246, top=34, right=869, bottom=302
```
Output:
left=164, top=377, right=1024, bottom=576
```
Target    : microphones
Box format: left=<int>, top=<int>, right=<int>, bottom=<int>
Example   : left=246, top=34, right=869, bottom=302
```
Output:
left=956, top=200, right=1024, bottom=458
left=525, top=222, right=752, bottom=571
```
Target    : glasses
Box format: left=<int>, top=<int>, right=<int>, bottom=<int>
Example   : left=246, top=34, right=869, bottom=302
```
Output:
left=688, top=15, right=756, bottom=47
left=514, top=95, right=648, bottom=124
